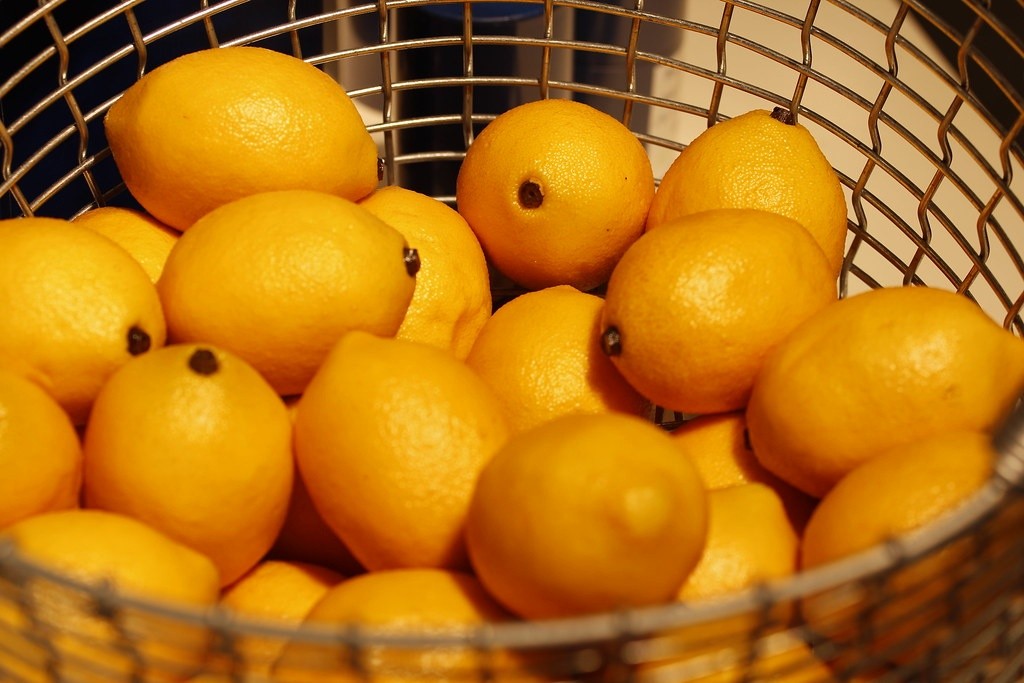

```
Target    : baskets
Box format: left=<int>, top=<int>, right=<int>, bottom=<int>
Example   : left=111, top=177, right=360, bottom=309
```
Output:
left=0, top=1, right=1023, bottom=681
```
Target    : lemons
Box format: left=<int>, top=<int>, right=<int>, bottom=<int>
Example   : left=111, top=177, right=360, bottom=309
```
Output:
left=0, top=44, right=1024, bottom=683
